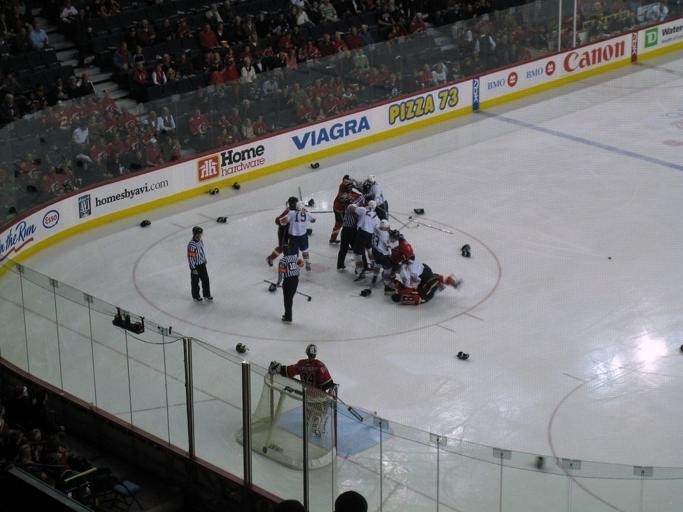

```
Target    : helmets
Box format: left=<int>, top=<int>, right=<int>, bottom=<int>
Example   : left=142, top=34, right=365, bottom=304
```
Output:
left=368, top=200, right=376, bottom=207
left=193, top=227, right=203, bottom=233
left=289, top=198, right=305, bottom=211
left=306, top=345, right=317, bottom=355
left=369, top=174, right=376, bottom=183
left=380, top=219, right=388, bottom=228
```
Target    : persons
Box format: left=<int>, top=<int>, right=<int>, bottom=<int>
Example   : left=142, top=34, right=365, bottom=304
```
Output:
left=0, top=382, right=367, bottom=512
left=1, top=1, right=285, bottom=229
left=267, top=175, right=461, bottom=322
left=284, top=1, right=560, bottom=124
left=558, top=1, right=681, bottom=51
left=187, top=226, right=214, bottom=303
left=269, top=343, right=335, bottom=434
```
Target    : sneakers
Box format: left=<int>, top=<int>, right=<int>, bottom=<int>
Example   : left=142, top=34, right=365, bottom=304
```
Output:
left=330, top=240, right=395, bottom=291
left=268, top=256, right=273, bottom=266
left=305, top=262, right=311, bottom=271
left=282, top=316, right=292, bottom=321
left=193, top=296, right=213, bottom=300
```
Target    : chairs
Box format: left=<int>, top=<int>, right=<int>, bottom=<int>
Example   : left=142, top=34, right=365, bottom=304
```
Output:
left=103, top=468, right=144, bottom=510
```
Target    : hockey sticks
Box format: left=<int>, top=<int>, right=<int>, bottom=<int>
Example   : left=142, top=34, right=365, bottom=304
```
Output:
left=337, top=396, right=377, bottom=423
left=264, top=279, right=311, bottom=303
left=388, top=213, right=419, bottom=229
left=352, top=188, right=366, bottom=206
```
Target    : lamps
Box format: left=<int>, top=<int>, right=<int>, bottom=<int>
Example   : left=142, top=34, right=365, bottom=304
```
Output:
left=111, top=309, right=145, bottom=336
